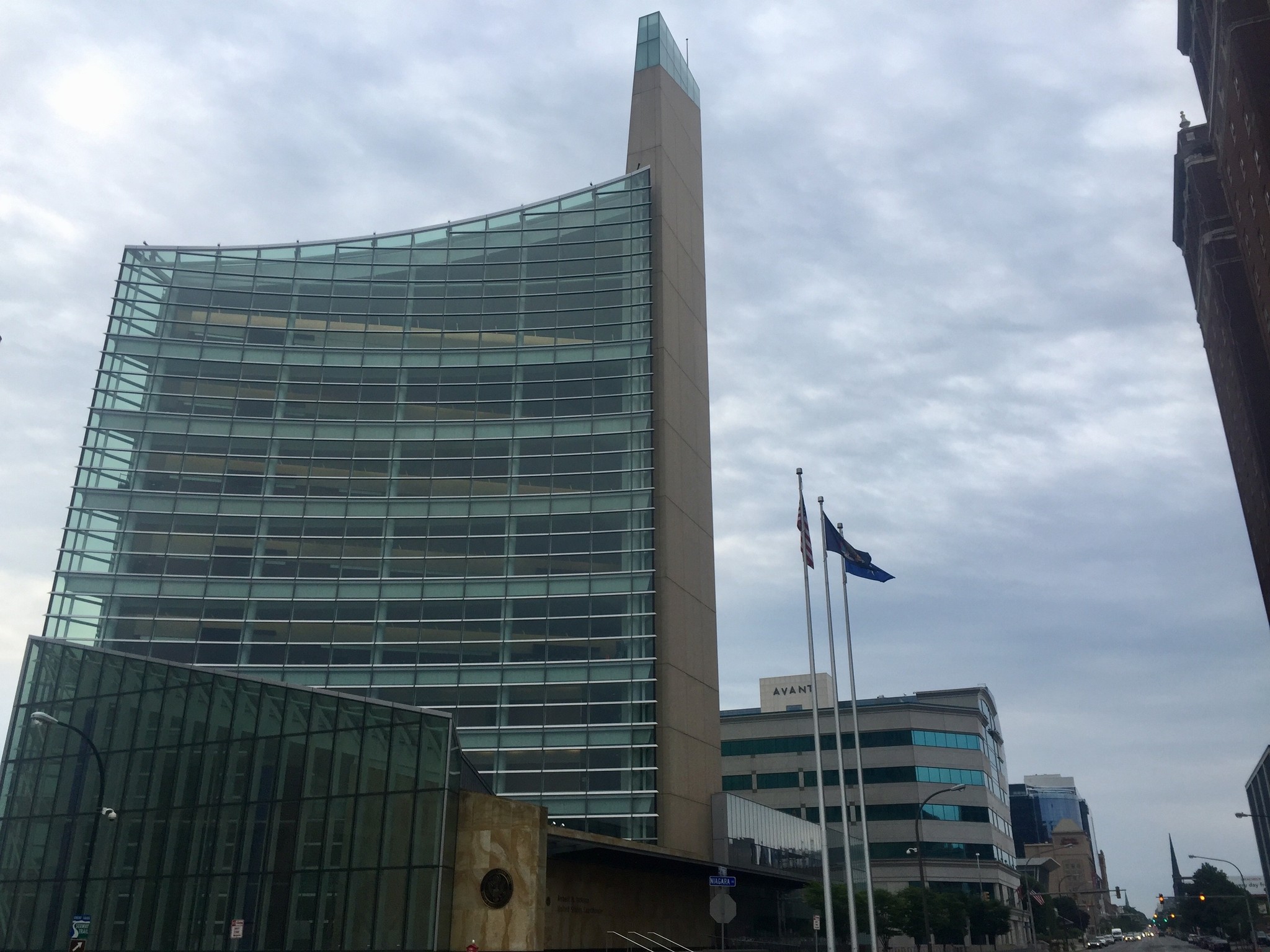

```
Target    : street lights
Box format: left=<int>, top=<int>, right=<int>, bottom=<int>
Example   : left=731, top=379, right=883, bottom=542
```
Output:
left=976, top=853, right=985, bottom=900
left=32, top=712, right=105, bottom=926
left=1058, top=874, right=1080, bottom=952
left=1025, top=843, right=1073, bottom=952
left=906, top=847, right=933, bottom=952
left=915, top=783, right=966, bottom=952
left=1188, top=853, right=1260, bottom=952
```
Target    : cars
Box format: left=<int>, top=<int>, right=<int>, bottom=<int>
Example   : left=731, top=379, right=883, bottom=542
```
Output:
left=1087, top=928, right=1155, bottom=949
left=1249, top=931, right=1270, bottom=947
left=1166, top=930, right=1231, bottom=952
left=1159, top=932, right=1165, bottom=938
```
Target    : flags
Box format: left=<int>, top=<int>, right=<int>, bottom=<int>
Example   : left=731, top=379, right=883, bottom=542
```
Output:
left=797, top=486, right=814, bottom=569
left=824, top=511, right=880, bottom=572
left=844, top=562, right=896, bottom=583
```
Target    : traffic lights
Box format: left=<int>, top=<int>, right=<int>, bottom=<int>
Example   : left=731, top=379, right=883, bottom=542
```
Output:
left=1158, top=893, right=1165, bottom=905
left=1199, top=892, right=1206, bottom=903
left=1152, top=913, right=1176, bottom=927
